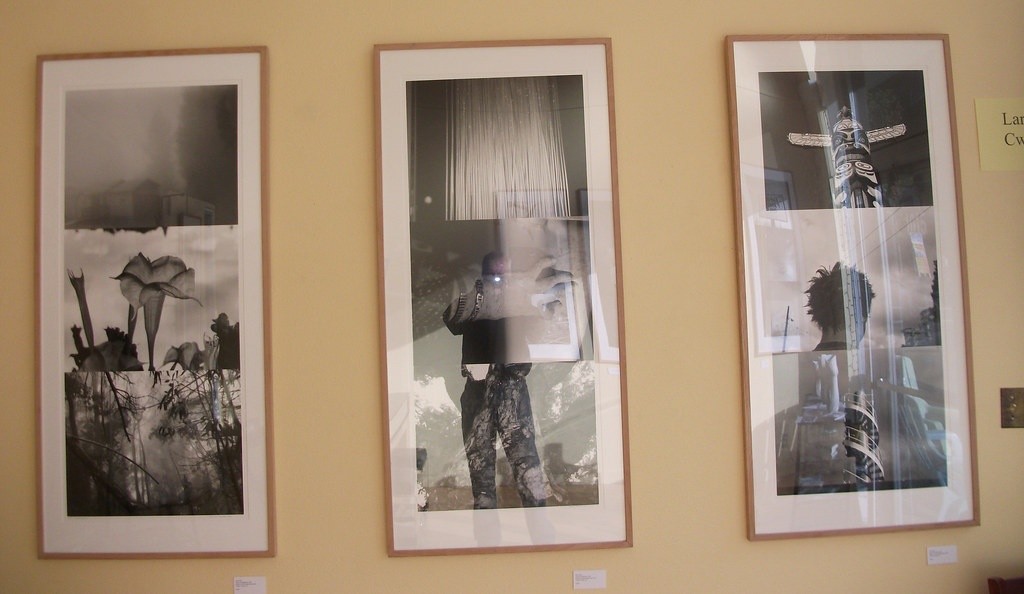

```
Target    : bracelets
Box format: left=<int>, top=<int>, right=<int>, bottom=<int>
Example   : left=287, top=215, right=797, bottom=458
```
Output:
left=448, top=270, right=484, bottom=333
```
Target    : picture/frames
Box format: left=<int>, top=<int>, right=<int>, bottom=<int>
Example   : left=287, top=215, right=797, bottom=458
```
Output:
left=723, top=33, right=984, bottom=542
left=32, top=44, right=279, bottom=562
left=372, top=36, right=635, bottom=559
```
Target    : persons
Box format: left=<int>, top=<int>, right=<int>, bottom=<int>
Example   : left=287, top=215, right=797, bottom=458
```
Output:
left=799, top=263, right=876, bottom=351
left=442, top=252, right=554, bottom=548
left=410, top=246, right=573, bottom=340
